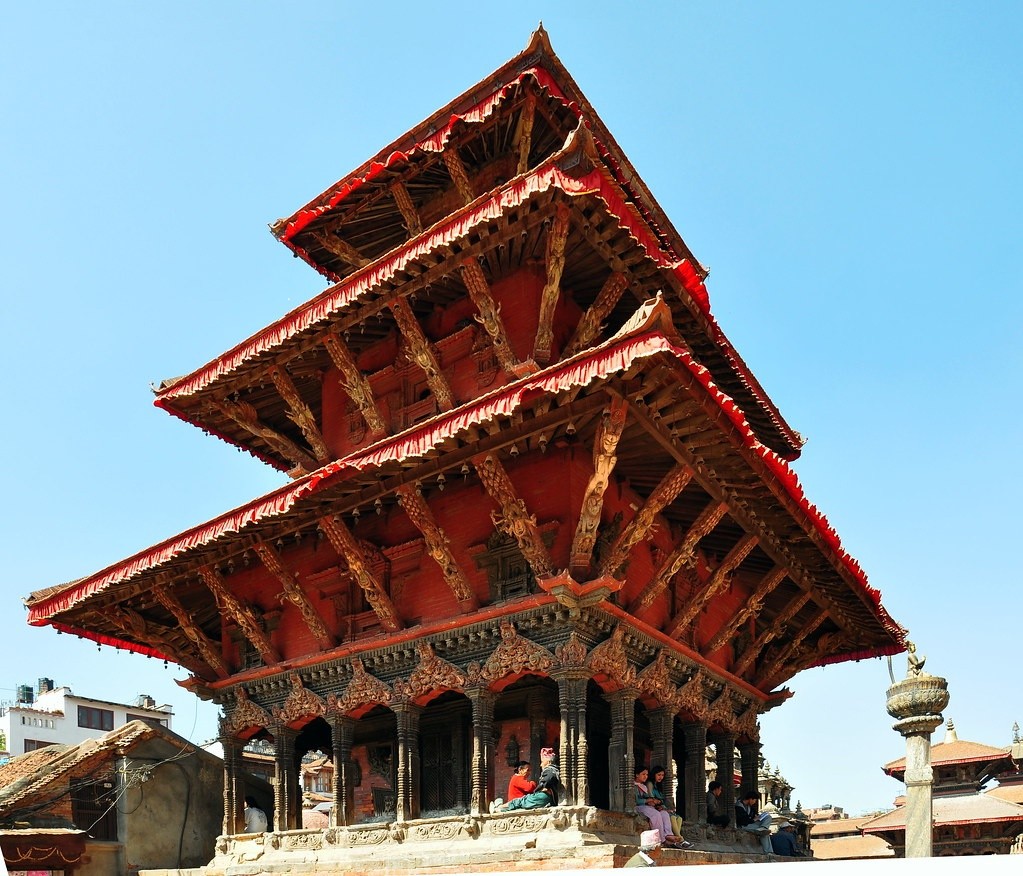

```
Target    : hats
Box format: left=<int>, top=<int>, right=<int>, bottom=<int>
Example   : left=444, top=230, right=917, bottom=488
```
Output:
left=780, top=822, right=795, bottom=828
left=541, top=748, right=556, bottom=761
left=640, top=829, right=661, bottom=847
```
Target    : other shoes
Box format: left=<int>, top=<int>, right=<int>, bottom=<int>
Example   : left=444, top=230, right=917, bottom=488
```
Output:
left=495, top=798, right=503, bottom=807
left=489, top=801, right=495, bottom=815
left=766, top=852, right=780, bottom=856
left=757, top=828, right=772, bottom=836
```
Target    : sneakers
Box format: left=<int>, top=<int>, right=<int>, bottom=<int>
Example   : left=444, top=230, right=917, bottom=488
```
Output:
left=673, top=841, right=694, bottom=848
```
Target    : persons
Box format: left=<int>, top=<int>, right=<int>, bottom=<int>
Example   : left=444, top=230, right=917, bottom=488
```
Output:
left=624, top=828, right=662, bottom=868
left=507, top=760, right=536, bottom=801
left=734, top=790, right=778, bottom=856
left=906, top=641, right=931, bottom=678
left=646, top=766, right=696, bottom=850
left=773, top=822, right=807, bottom=857
left=705, top=781, right=732, bottom=831
left=632, top=766, right=682, bottom=848
left=489, top=747, right=559, bottom=815
